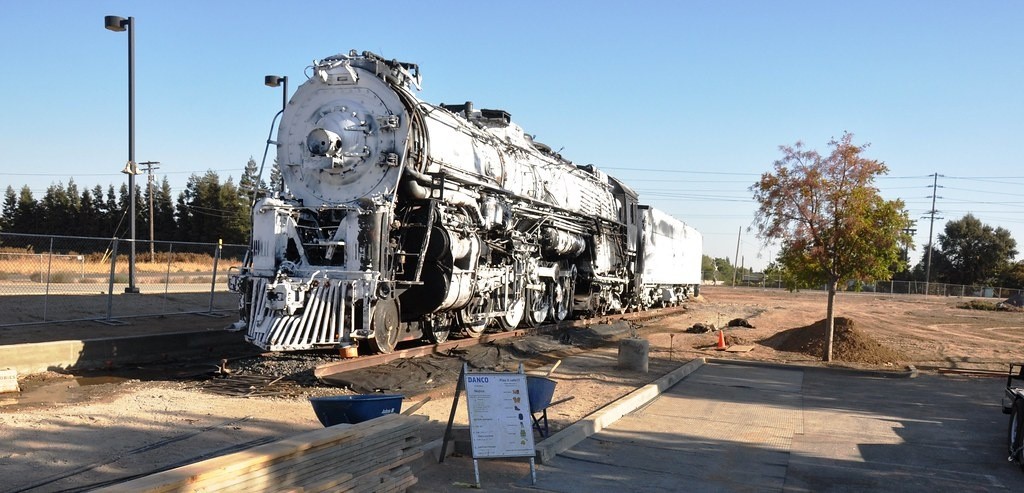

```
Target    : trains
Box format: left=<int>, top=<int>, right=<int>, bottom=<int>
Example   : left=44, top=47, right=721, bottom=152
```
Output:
left=222, top=49, right=703, bottom=360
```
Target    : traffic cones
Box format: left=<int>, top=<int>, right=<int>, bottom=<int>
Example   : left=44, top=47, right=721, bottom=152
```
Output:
left=715, top=329, right=730, bottom=351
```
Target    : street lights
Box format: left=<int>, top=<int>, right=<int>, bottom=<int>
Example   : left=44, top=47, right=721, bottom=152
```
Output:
left=103, top=11, right=141, bottom=295
left=264, top=71, right=289, bottom=118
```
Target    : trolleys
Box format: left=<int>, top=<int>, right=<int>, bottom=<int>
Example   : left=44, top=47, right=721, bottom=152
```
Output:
left=507, top=360, right=576, bottom=439
left=307, top=392, right=431, bottom=429
